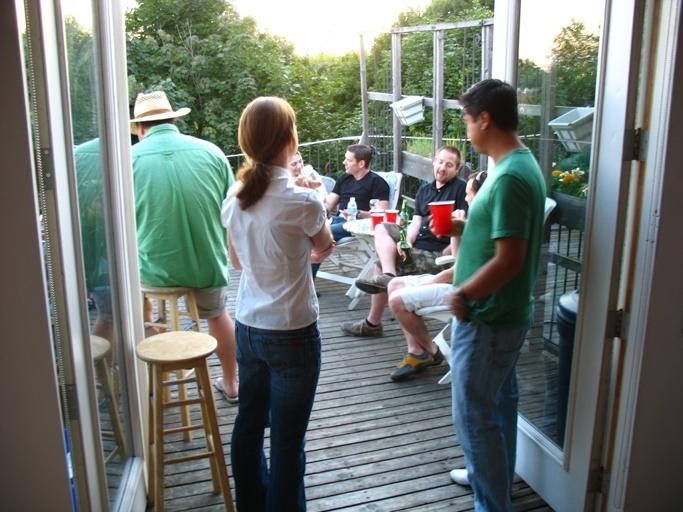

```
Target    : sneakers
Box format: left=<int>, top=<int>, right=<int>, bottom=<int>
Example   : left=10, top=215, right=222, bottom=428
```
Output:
left=390, top=352, right=434, bottom=381
left=429, top=345, right=445, bottom=365
left=355, top=274, right=394, bottom=293
left=341, top=320, right=383, bottom=337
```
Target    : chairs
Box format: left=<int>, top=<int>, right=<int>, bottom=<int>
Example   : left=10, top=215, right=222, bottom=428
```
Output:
left=320, top=176, right=340, bottom=219
left=416, top=195, right=557, bottom=386
left=318, top=172, right=402, bottom=300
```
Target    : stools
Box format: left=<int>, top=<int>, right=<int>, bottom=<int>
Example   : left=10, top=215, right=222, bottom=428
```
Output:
left=90, top=335, right=129, bottom=469
left=136, top=331, right=238, bottom=512
left=140, top=285, right=202, bottom=444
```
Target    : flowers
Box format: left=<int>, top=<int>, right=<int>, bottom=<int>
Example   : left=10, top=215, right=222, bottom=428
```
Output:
left=549, top=168, right=588, bottom=197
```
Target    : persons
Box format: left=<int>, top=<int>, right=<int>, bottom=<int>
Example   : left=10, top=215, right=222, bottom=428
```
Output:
left=309, top=143, right=391, bottom=299
left=382, top=165, right=493, bottom=385
left=338, top=144, right=473, bottom=339
left=426, top=76, right=550, bottom=512
left=74, top=136, right=127, bottom=415
left=285, top=148, right=327, bottom=199
left=217, top=93, right=336, bottom=512
left=129, top=88, right=245, bottom=408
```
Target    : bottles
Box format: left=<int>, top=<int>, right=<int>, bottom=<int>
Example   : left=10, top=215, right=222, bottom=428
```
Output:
left=396, top=230, right=414, bottom=266
left=346, top=197, right=356, bottom=227
left=399, top=200, right=408, bottom=228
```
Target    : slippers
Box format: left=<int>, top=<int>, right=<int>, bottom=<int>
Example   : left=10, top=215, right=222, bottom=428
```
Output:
left=215, top=375, right=239, bottom=402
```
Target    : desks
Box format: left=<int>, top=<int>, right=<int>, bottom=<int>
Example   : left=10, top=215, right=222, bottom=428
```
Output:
left=343, top=217, right=400, bottom=311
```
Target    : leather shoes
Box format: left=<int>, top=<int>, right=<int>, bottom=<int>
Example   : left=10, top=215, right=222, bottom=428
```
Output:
left=450, top=469, right=470, bottom=485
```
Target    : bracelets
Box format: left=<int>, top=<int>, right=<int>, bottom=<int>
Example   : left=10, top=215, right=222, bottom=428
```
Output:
left=453, top=286, right=470, bottom=307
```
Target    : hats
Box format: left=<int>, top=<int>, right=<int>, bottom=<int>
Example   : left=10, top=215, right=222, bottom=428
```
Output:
left=130, top=91, right=192, bottom=135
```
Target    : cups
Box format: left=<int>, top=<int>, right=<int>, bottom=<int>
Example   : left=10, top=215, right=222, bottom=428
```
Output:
left=369, top=209, right=383, bottom=214
left=385, top=209, right=398, bottom=223
left=428, top=200, right=454, bottom=235
left=370, top=213, right=384, bottom=229
